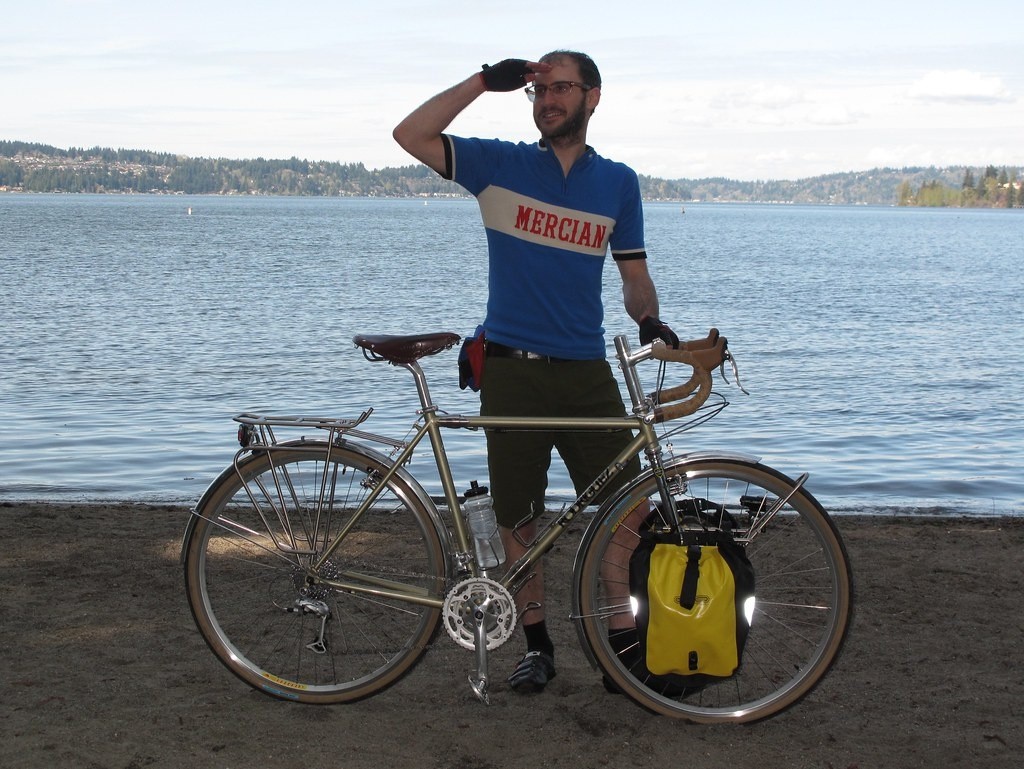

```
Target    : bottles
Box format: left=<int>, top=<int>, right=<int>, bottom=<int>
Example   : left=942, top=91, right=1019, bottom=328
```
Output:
left=463, top=480, right=506, bottom=569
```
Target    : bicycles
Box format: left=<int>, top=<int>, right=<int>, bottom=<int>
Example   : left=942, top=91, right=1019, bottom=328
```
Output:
left=179, top=328, right=855, bottom=725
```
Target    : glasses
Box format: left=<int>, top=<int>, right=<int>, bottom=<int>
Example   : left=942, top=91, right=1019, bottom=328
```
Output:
left=525, top=81, right=592, bottom=102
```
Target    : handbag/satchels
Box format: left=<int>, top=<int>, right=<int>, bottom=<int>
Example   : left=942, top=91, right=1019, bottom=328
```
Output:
left=627, top=498, right=756, bottom=678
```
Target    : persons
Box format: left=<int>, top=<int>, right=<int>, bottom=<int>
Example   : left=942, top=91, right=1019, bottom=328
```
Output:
left=393, top=51, right=678, bottom=691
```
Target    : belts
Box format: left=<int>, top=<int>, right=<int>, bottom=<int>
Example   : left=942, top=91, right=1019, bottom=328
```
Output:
left=484, top=340, right=544, bottom=361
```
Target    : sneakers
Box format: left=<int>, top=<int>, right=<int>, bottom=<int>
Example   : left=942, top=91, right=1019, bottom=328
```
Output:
left=602, top=654, right=695, bottom=698
left=507, top=650, right=556, bottom=694
